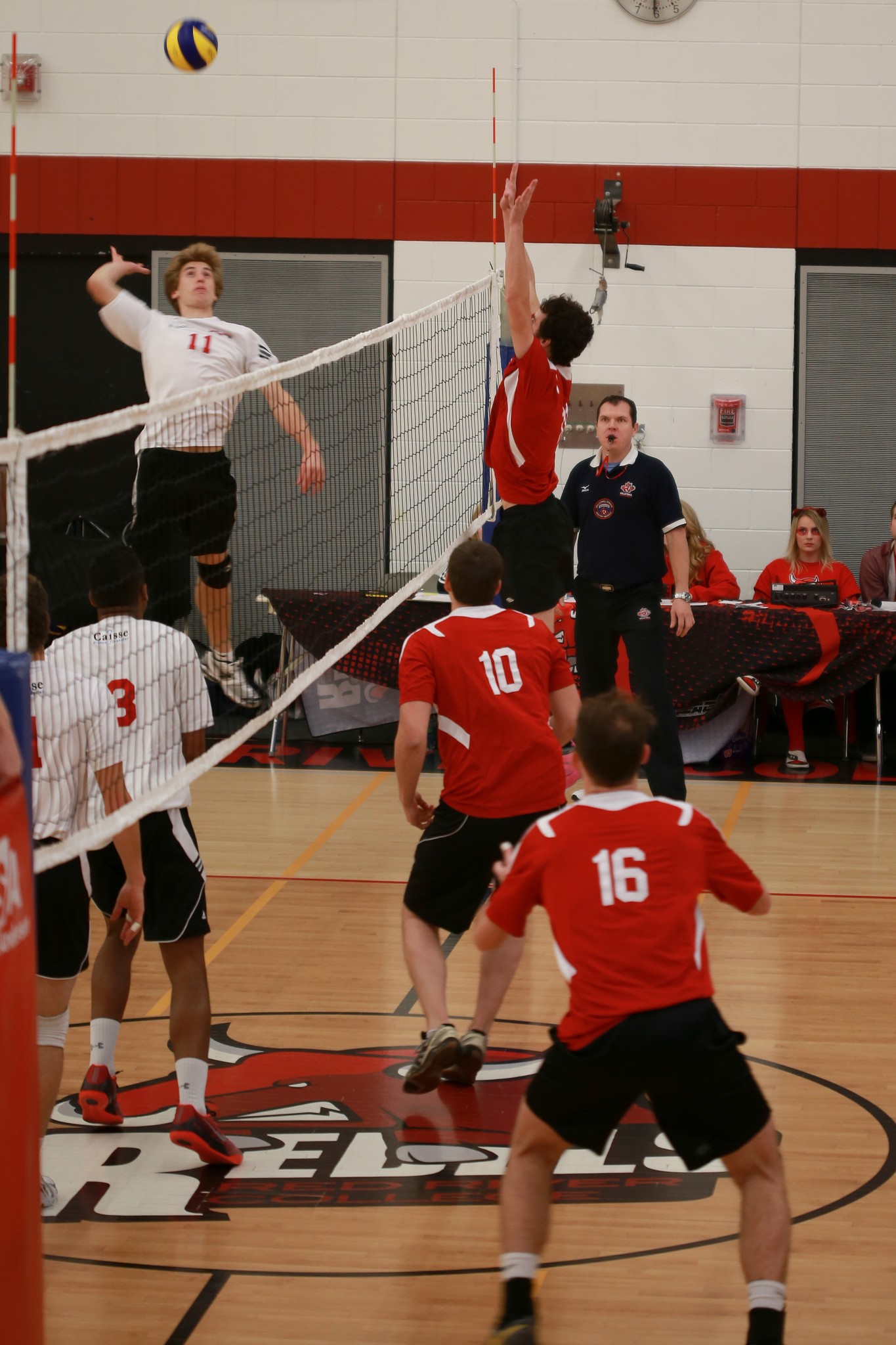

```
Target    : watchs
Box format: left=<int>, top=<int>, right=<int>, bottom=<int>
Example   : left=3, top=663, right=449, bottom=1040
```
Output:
left=674, top=592, right=692, bottom=602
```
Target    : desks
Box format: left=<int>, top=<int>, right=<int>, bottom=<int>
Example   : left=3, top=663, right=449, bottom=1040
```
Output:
left=255, top=589, right=896, bottom=777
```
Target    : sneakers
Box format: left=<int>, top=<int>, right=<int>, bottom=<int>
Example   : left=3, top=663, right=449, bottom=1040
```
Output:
left=77, top=1063, right=124, bottom=1126
left=168, top=1103, right=243, bottom=1166
left=440, top=1028, right=488, bottom=1084
left=404, top=1023, right=461, bottom=1094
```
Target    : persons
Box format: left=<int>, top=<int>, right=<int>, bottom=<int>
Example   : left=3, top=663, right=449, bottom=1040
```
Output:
left=0, top=153, right=896, bottom=1242
left=469, top=689, right=790, bottom=1345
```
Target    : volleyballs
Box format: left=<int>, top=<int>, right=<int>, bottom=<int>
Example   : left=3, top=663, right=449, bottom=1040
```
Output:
left=164, top=19, right=219, bottom=73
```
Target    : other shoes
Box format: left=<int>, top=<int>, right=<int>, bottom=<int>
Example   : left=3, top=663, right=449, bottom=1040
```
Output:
left=39, top=1180, right=58, bottom=1209
left=786, top=751, right=811, bottom=770
left=489, top=1318, right=535, bottom=1344
left=200, top=649, right=260, bottom=706
left=736, top=674, right=761, bottom=697
left=571, top=788, right=584, bottom=801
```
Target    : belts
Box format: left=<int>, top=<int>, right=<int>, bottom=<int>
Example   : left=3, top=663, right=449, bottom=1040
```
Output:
left=594, top=582, right=637, bottom=593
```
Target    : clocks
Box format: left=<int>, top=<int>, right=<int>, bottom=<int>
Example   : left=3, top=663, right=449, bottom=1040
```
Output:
left=616, top=0, right=696, bottom=24
